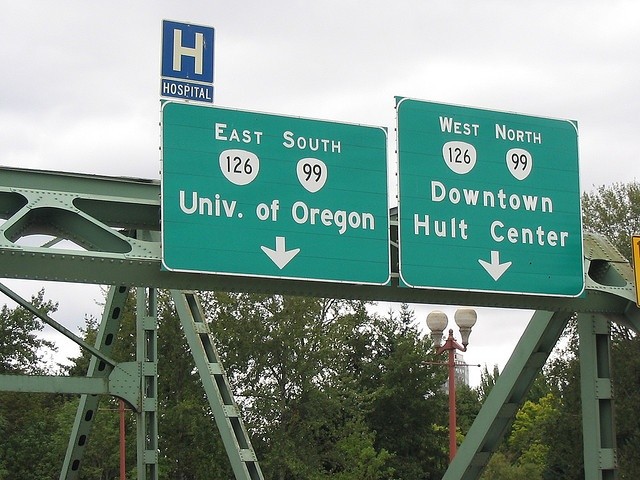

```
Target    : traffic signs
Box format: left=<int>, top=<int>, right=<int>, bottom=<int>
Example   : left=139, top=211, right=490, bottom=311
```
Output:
left=159, top=18, right=215, bottom=103
left=396, top=98, right=585, bottom=298
left=158, top=99, right=392, bottom=286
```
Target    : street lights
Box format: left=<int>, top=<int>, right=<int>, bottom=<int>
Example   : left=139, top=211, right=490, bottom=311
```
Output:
left=421, top=309, right=481, bottom=463
